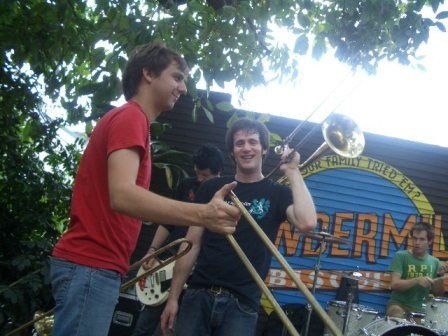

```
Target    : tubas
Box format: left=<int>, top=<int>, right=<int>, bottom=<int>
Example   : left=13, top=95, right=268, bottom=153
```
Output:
left=2, top=238, right=193, bottom=336
left=263, top=80, right=366, bottom=185
left=223, top=189, right=343, bottom=336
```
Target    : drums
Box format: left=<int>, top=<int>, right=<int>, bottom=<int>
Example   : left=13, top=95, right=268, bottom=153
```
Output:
left=355, top=315, right=437, bottom=336
left=419, top=296, right=448, bottom=336
left=323, top=300, right=380, bottom=336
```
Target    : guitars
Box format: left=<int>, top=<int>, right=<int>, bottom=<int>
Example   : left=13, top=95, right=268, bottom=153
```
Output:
left=134, top=254, right=197, bottom=307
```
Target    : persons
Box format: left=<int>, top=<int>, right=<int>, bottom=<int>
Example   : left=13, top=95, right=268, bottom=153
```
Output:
left=162, top=118, right=318, bottom=335
left=385, top=221, right=448, bottom=327
left=127, top=146, right=225, bottom=335
left=49, top=42, right=244, bottom=336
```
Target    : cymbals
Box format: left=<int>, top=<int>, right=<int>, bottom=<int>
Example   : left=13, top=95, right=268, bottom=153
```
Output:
left=294, top=230, right=354, bottom=246
left=326, top=271, right=386, bottom=286
